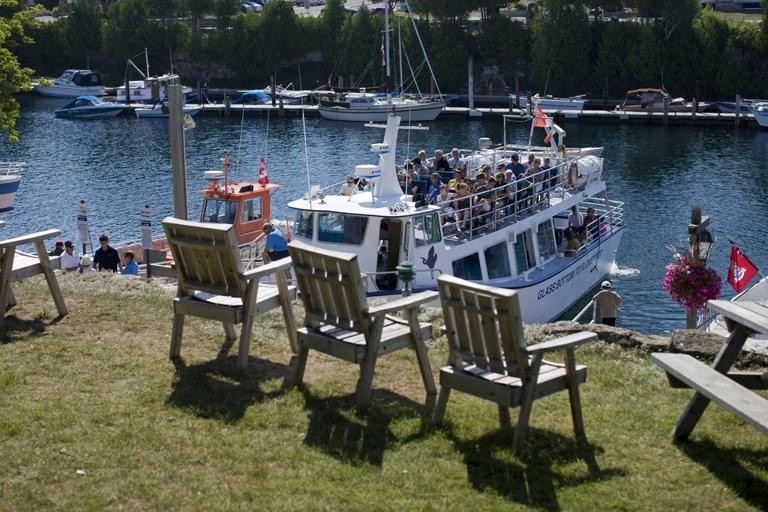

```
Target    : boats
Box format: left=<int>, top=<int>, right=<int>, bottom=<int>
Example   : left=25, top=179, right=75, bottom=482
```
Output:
left=507, top=94, right=588, bottom=110
left=230, top=81, right=309, bottom=105
left=715, top=102, right=750, bottom=114
left=0, top=161, right=24, bottom=214
left=55, top=95, right=125, bottom=120
left=36, top=68, right=105, bottom=99
left=136, top=102, right=200, bottom=119
left=84, top=180, right=301, bottom=287
left=616, top=88, right=710, bottom=112
left=698, top=274, right=768, bottom=342
left=743, top=98, right=768, bottom=127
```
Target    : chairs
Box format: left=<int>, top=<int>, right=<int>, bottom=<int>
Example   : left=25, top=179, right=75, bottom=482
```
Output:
left=430, top=272, right=597, bottom=451
left=287, top=239, right=437, bottom=411
left=161, top=215, right=294, bottom=373
left=0, top=220, right=69, bottom=324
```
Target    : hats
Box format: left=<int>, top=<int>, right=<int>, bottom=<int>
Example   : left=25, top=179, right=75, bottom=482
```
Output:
left=80, top=254, right=90, bottom=265
left=601, top=281, right=611, bottom=288
left=65, top=241, right=75, bottom=247
left=512, top=155, right=518, bottom=161
left=55, top=241, right=63, bottom=247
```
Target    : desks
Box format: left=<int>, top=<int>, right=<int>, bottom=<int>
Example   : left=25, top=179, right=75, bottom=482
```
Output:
left=671, top=297, right=768, bottom=452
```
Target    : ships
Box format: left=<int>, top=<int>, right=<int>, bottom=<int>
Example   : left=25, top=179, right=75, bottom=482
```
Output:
left=288, top=95, right=626, bottom=335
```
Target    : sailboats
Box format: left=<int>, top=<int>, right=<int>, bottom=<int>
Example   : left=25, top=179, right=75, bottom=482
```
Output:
left=315, top=0, right=447, bottom=122
left=116, top=48, right=208, bottom=104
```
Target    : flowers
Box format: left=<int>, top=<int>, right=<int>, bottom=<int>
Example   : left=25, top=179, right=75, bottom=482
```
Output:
left=662, top=244, right=722, bottom=312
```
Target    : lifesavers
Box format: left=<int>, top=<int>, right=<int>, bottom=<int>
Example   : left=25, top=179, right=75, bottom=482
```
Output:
left=465, top=155, right=491, bottom=176
left=564, top=155, right=603, bottom=185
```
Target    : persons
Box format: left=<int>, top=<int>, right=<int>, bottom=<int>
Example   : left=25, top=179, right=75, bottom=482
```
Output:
left=593, top=281, right=623, bottom=327
left=339, top=149, right=606, bottom=274
left=59, top=241, right=80, bottom=269
left=49, top=241, right=64, bottom=256
left=261, top=223, right=290, bottom=266
left=122, top=250, right=138, bottom=275
left=93, top=234, right=123, bottom=273
left=76, top=254, right=97, bottom=273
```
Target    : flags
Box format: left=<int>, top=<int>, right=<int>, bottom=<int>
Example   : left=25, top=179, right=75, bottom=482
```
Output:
left=534, top=107, right=553, bottom=128
left=727, top=244, right=759, bottom=293
left=258, top=158, right=269, bottom=185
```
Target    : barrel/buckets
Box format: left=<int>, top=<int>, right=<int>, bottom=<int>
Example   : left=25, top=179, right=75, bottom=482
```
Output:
left=573, top=154, right=601, bottom=188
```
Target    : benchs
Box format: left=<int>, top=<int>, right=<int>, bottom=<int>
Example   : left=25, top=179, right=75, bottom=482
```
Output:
left=651, top=351, right=768, bottom=433
left=397, top=154, right=557, bottom=230
